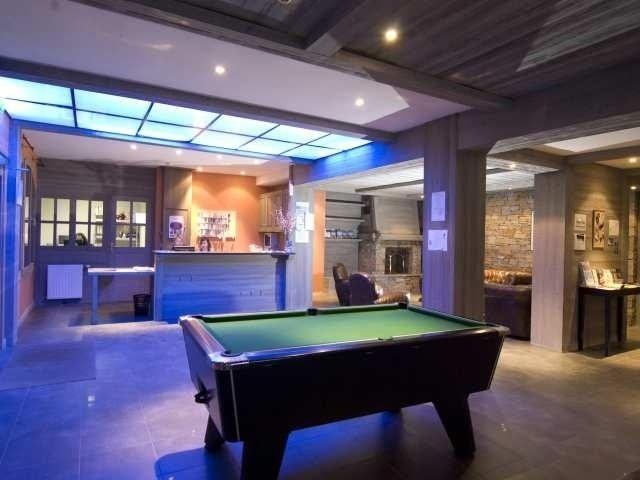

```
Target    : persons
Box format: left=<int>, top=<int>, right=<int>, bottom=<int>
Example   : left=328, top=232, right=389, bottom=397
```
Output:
left=199, top=237, right=215, bottom=251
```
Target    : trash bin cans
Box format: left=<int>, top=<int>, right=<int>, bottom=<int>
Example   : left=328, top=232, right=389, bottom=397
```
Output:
left=135, top=294, right=151, bottom=319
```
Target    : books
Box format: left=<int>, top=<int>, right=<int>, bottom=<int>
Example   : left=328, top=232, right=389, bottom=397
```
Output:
left=578, top=261, right=640, bottom=290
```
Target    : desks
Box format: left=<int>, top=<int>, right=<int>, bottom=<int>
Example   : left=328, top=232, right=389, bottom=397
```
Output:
left=575, top=283, right=640, bottom=359
left=179, top=305, right=514, bottom=480
left=86, top=266, right=153, bottom=325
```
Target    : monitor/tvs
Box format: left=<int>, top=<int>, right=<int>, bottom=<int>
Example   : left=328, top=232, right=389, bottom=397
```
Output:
left=172, top=246, right=194, bottom=251
left=263, top=232, right=278, bottom=247
